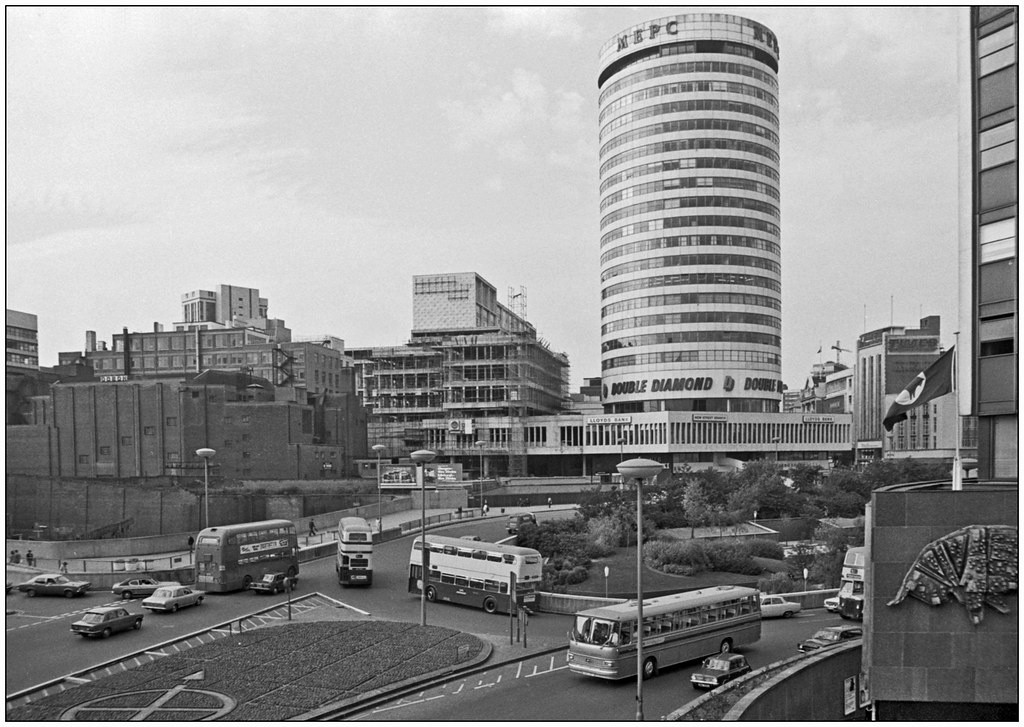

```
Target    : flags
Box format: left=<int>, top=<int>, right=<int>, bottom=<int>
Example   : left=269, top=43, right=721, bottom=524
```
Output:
left=882, top=344, right=954, bottom=431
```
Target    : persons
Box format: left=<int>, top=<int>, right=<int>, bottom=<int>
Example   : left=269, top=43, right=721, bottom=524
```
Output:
left=60, top=562, right=68, bottom=573
left=483, top=504, right=488, bottom=516
left=9, top=549, right=21, bottom=563
left=375, top=518, right=380, bottom=528
left=547, top=496, right=552, bottom=508
left=26, top=550, right=33, bottom=565
left=484, top=498, right=488, bottom=504
left=861, top=690, right=865, bottom=703
left=849, top=679, right=854, bottom=691
left=309, top=518, right=315, bottom=536
left=608, top=630, right=619, bottom=646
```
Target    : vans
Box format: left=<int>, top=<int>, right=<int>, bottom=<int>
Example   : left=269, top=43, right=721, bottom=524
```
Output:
left=506, top=512, right=537, bottom=535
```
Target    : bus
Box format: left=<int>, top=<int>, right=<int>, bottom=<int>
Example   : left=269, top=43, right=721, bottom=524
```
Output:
left=408, top=533, right=543, bottom=616
left=335, top=516, right=374, bottom=587
left=836, top=547, right=865, bottom=621
left=194, top=519, right=299, bottom=593
left=566, top=584, right=762, bottom=682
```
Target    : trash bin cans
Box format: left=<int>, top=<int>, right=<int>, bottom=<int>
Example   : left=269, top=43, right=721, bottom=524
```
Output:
left=454, top=510, right=460, bottom=518
left=142, top=559, right=154, bottom=569
left=467, top=510, right=473, bottom=516
left=601, top=475, right=612, bottom=482
left=113, top=559, right=124, bottom=571
left=125, top=558, right=138, bottom=571
left=462, top=511, right=468, bottom=517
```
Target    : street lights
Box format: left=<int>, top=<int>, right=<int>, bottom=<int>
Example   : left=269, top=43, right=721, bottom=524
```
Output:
left=475, top=440, right=486, bottom=516
left=616, top=458, right=668, bottom=721
left=617, top=438, right=627, bottom=491
left=410, top=449, right=437, bottom=627
left=772, top=437, right=781, bottom=462
left=371, top=443, right=387, bottom=542
left=195, top=447, right=217, bottom=527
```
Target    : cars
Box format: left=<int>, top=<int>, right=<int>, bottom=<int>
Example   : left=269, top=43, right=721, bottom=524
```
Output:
left=111, top=574, right=182, bottom=601
left=753, top=594, right=802, bottom=619
left=249, top=571, right=298, bottom=595
left=70, top=605, right=144, bottom=639
left=459, top=534, right=484, bottom=542
left=796, top=625, right=862, bottom=653
left=824, top=592, right=840, bottom=613
left=19, top=573, right=92, bottom=599
left=140, top=585, right=206, bottom=614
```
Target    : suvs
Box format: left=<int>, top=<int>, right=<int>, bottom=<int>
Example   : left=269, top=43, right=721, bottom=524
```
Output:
left=690, top=652, right=752, bottom=690
